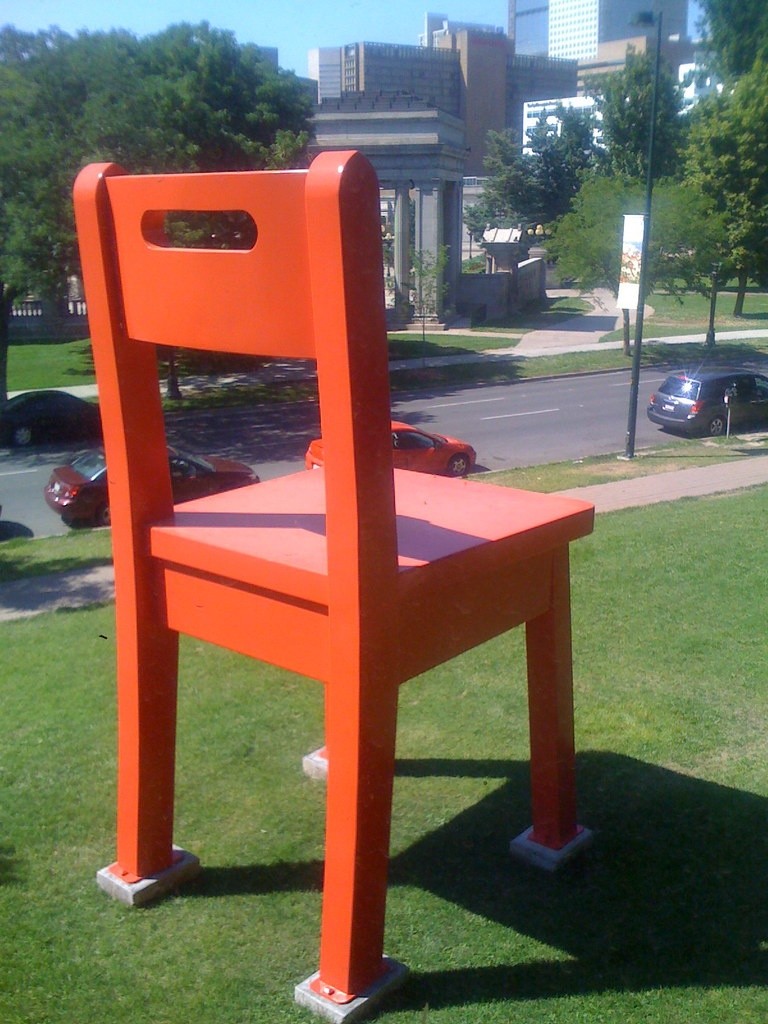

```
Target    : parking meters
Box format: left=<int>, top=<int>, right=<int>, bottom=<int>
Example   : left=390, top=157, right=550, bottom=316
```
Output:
left=724, top=388, right=738, bottom=439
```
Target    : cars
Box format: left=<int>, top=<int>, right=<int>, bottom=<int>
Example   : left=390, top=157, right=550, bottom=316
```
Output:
left=0, top=390, right=102, bottom=445
left=43, top=445, right=260, bottom=528
left=305, top=419, right=475, bottom=477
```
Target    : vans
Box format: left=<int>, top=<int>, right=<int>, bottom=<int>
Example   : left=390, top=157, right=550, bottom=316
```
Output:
left=647, top=367, right=768, bottom=436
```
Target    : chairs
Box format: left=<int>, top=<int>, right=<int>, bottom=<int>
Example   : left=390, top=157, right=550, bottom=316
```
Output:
left=65, top=146, right=595, bottom=1024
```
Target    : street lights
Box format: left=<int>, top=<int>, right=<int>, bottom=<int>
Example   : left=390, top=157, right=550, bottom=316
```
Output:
left=624, top=9, right=663, bottom=458
